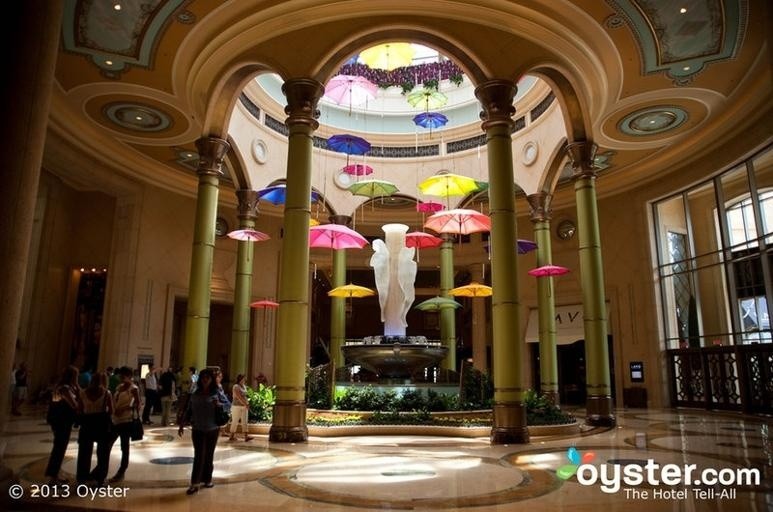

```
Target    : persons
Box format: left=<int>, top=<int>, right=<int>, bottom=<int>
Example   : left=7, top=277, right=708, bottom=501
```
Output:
left=11, top=361, right=32, bottom=417
left=11, top=363, right=18, bottom=393
left=44, top=362, right=255, bottom=495
left=679, top=339, right=692, bottom=384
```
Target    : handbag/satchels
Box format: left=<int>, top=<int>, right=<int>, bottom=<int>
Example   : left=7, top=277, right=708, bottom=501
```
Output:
left=131, top=418, right=144, bottom=441
left=215, top=406, right=229, bottom=426
left=47, top=399, right=75, bottom=426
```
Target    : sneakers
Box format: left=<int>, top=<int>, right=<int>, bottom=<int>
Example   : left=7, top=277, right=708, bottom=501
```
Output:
left=47, top=476, right=125, bottom=489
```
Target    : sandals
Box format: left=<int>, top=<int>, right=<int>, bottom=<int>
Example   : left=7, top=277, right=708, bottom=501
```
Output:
left=206, top=481, right=214, bottom=488
left=245, top=437, right=253, bottom=441
left=187, top=486, right=198, bottom=495
left=222, top=434, right=237, bottom=440
